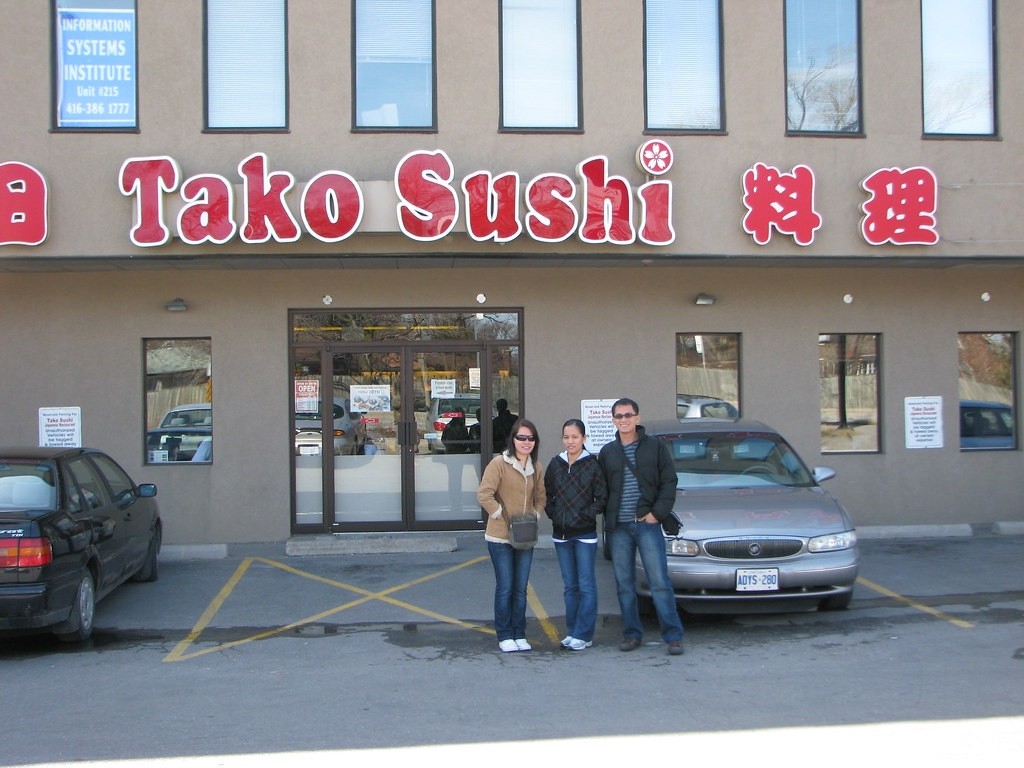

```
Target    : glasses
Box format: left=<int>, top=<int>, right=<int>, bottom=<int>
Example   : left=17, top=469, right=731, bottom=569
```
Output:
left=514, top=434, right=536, bottom=442
left=613, top=412, right=636, bottom=419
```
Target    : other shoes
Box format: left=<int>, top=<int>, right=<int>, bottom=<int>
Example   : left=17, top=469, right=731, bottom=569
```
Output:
left=619, top=637, right=642, bottom=651
left=499, top=638, right=518, bottom=652
left=514, top=639, right=531, bottom=651
left=668, top=640, right=683, bottom=655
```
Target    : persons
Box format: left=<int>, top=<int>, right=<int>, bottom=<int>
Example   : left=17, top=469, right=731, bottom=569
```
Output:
left=476, top=418, right=547, bottom=653
left=971, top=411, right=990, bottom=435
left=440, top=398, right=519, bottom=517
left=544, top=418, right=609, bottom=651
left=598, top=396, right=683, bottom=655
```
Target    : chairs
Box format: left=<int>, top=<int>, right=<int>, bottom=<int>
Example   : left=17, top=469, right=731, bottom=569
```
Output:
left=970, top=417, right=991, bottom=436
left=162, top=443, right=169, bottom=460
left=170, top=418, right=187, bottom=427
left=203, top=417, right=212, bottom=425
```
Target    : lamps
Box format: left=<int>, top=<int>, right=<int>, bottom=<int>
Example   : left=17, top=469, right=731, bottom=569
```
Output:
left=162, top=296, right=188, bottom=313
left=688, top=293, right=726, bottom=311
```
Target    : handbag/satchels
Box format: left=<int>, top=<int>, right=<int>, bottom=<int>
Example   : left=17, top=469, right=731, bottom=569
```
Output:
left=509, top=512, right=539, bottom=551
left=659, top=511, right=684, bottom=541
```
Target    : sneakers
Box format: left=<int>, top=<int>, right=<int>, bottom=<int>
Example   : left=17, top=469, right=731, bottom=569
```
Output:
left=559, top=635, right=593, bottom=650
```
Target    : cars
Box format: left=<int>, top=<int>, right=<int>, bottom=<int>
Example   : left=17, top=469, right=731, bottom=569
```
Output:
left=602, top=420, right=861, bottom=622
left=292, top=398, right=368, bottom=456
left=0, top=447, right=164, bottom=647
left=676, top=394, right=737, bottom=421
left=423, top=392, right=497, bottom=454
left=960, top=399, right=1015, bottom=448
left=146, top=403, right=214, bottom=462
